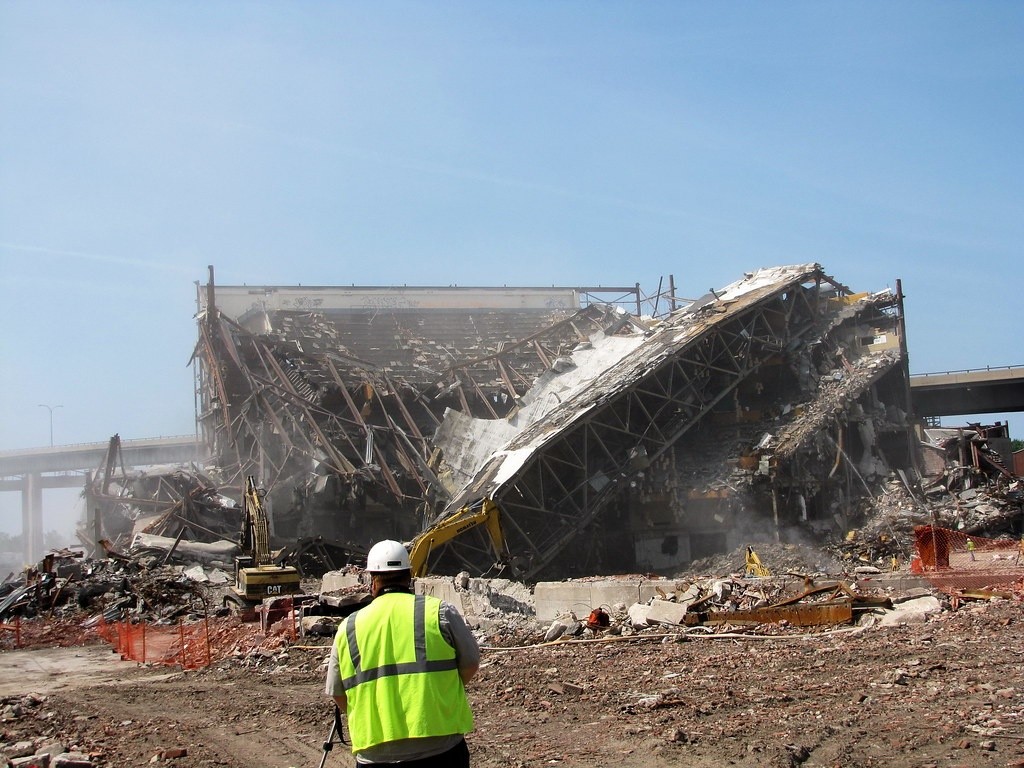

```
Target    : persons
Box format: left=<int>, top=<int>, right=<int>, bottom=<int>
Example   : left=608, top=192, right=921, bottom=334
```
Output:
left=964, top=538, right=977, bottom=561
left=324, top=539, right=478, bottom=767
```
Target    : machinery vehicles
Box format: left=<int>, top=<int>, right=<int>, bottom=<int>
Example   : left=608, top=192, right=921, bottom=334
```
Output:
left=407, top=495, right=523, bottom=595
left=220, top=474, right=306, bottom=624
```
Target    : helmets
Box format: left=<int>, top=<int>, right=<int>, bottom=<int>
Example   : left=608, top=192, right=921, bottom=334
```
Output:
left=967, top=538, right=971, bottom=541
left=364, top=539, right=413, bottom=572
